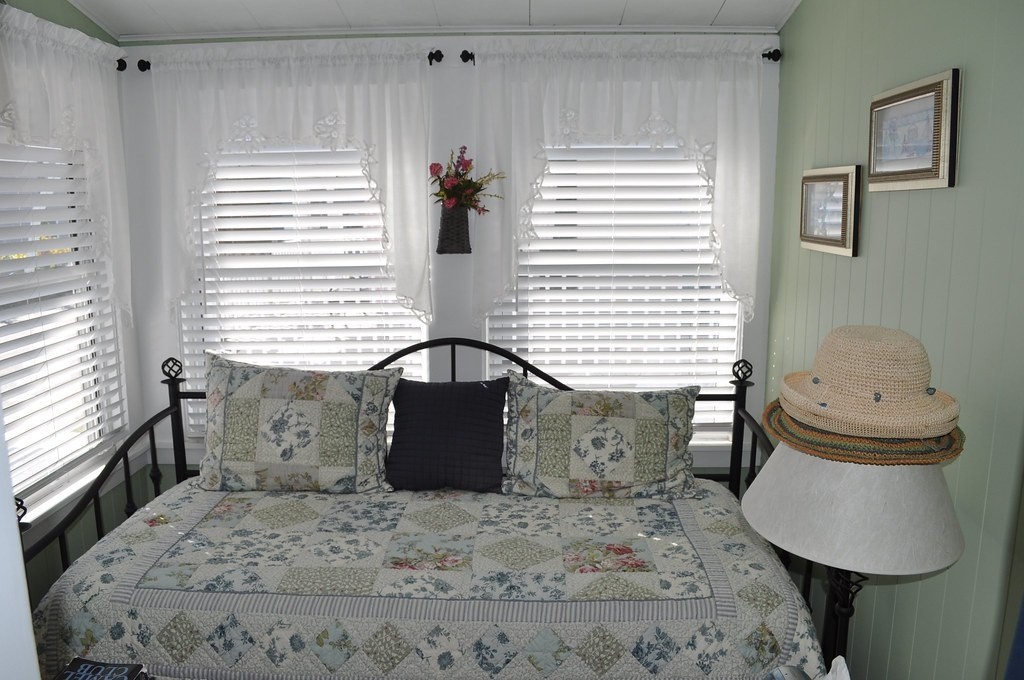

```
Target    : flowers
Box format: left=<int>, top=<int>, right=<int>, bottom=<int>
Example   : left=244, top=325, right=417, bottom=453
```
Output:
left=428, top=146, right=507, bottom=215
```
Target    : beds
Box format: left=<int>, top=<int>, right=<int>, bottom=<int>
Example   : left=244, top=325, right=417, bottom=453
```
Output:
left=12, top=338, right=830, bottom=680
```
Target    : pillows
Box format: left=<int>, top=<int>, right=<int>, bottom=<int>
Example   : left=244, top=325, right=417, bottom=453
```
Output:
left=500, top=367, right=705, bottom=501
left=198, top=351, right=403, bottom=494
left=384, top=376, right=511, bottom=493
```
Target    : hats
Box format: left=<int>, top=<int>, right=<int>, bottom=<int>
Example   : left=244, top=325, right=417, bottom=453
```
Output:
left=762, top=325, right=967, bottom=466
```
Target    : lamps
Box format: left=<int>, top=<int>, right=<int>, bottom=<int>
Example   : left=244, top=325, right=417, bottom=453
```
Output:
left=742, top=441, right=965, bottom=680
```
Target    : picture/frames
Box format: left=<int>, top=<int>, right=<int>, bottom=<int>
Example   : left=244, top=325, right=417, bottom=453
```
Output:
left=865, top=68, right=960, bottom=191
left=799, top=163, right=862, bottom=258
